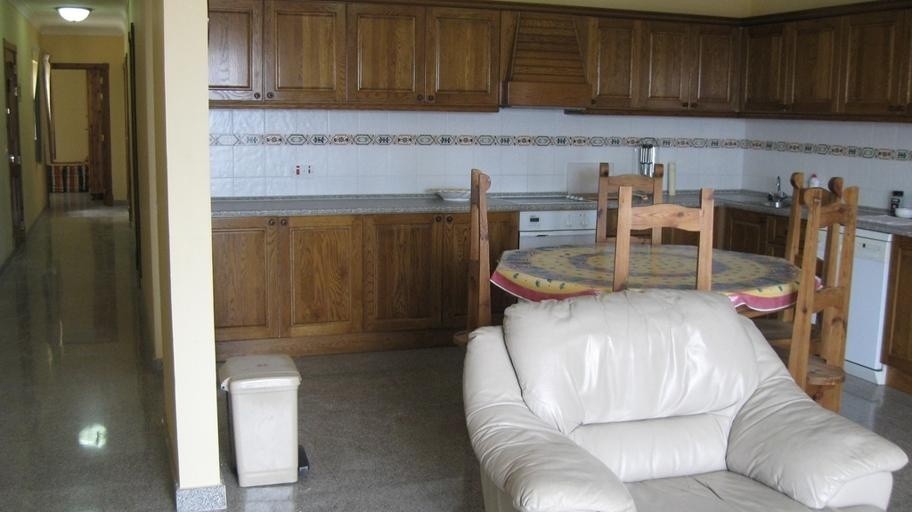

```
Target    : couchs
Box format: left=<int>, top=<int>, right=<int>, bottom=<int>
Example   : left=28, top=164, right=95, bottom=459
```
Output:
left=462, top=287, right=908, bottom=512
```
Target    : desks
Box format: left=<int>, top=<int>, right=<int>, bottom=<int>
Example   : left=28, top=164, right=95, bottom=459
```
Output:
left=493, top=238, right=820, bottom=323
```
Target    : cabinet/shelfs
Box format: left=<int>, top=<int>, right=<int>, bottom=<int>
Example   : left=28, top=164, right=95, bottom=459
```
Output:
left=878, top=235, right=912, bottom=397
left=342, top=0, right=503, bottom=113
left=721, top=204, right=825, bottom=328
left=351, top=213, right=520, bottom=349
left=635, top=9, right=742, bottom=117
left=206, top=0, right=348, bottom=108
left=740, top=6, right=841, bottom=124
left=836, top=1, right=910, bottom=126
left=569, top=9, right=641, bottom=117
left=210, top=217, right=364, bottom=361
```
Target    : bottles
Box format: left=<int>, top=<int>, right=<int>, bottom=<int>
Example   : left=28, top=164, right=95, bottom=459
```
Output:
left=889, top=191, right=903, bottom=216
left=809, top=174, right=819, bottom=188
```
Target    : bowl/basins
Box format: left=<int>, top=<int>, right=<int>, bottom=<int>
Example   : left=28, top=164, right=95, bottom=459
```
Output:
left=893, top=207, right=912, bottom=219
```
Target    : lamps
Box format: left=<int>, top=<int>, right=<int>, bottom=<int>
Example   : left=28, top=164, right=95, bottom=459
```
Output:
left=56, top=6, right=92, bottom=22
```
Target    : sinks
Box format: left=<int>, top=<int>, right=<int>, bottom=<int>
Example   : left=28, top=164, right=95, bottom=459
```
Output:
left=489, top=193, right=586, bottom=203
left=764, top=199, right=791, bottom=210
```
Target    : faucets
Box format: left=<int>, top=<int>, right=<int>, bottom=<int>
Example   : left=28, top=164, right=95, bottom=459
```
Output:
left=776, top=175, right=781, bottom=192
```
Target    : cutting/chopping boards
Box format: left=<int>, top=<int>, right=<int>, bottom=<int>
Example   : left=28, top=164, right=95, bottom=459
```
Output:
left=437, top=192, right=470, bottom=201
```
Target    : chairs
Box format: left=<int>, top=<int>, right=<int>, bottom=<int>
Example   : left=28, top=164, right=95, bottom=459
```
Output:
left=768, top=180, right=862, bottom=418
left=593, top=161, right=665, bottom=245
left=470, top=168, right=501, bottom=334
left=740, top=172, right=846, bottom=352
left=606, top=180, right=718, bottom=289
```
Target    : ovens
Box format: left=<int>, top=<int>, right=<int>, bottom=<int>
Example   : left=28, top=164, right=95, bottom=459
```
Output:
left=516, top=231, right=596, bottom=304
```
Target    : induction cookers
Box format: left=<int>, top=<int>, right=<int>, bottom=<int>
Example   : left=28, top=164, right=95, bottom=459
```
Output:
left=493, top=194, right=597, bottom=231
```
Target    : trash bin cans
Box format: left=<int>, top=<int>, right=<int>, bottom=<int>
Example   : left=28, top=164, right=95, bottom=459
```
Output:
left=218, top=353, right=310, bottom=488
